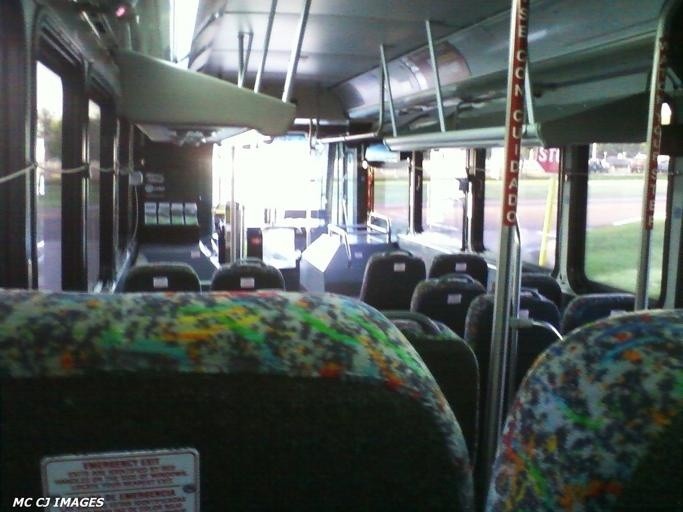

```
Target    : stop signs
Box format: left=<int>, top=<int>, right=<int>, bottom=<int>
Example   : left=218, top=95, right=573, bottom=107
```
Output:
left=536, top=144, right=560, bottom=174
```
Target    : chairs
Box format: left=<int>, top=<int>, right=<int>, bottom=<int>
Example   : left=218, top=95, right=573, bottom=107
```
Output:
left=0, top=248, right=682, bottom=512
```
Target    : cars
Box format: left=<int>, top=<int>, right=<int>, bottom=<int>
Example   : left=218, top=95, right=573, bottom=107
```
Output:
left=587, top=150, right=670, bottom=174
